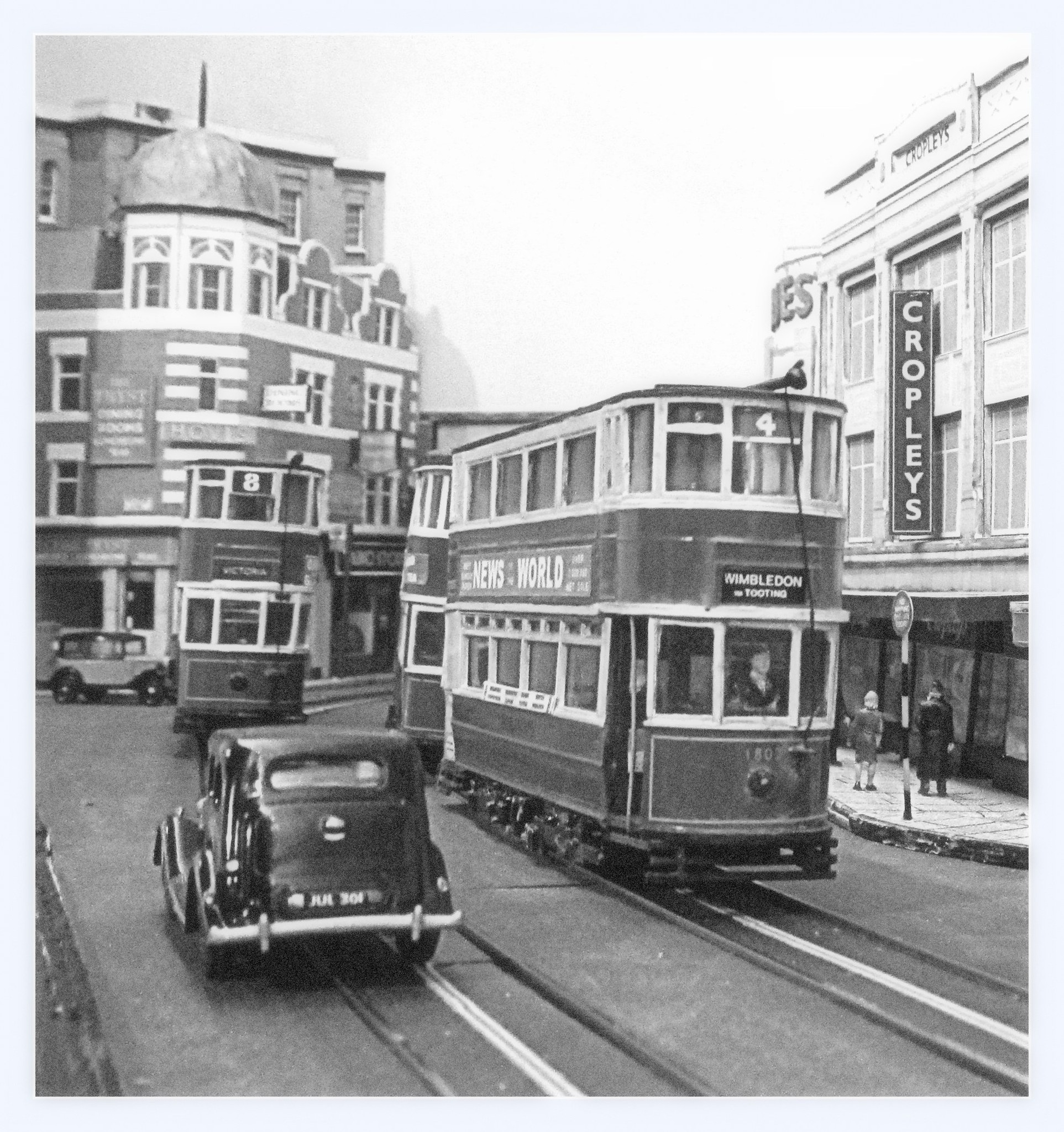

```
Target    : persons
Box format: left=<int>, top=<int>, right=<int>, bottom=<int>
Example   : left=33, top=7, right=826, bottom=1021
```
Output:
left=829, top=680, right=852, bottom=764
left=915, top=681, right=956, bottom=797
left=725, top=642, right=787, bottom=715
left=847, top=691, right=883, bottom=791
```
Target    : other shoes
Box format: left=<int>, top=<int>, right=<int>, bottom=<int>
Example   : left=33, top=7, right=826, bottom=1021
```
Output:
left=918, top=788, right=929, bottom=796
left=866, top=783, right=877, bottom=791
left=938, top=791, right=946, bottom=797
left=831, top=759, right=842, bottom=766
left=853, top=781, right=861, bottom=791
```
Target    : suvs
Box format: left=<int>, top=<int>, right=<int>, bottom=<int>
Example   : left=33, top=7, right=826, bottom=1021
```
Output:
left=152, top=723, right=462, bottom=979
left=48, top=627, right=181, bottom=707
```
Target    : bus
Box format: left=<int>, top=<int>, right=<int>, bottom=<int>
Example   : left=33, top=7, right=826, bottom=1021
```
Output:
left=383, top=455, right=454, bottom=775
left=167, top=577, right=316, bottom=735
left=435, top=381, right=853, bottom=890
